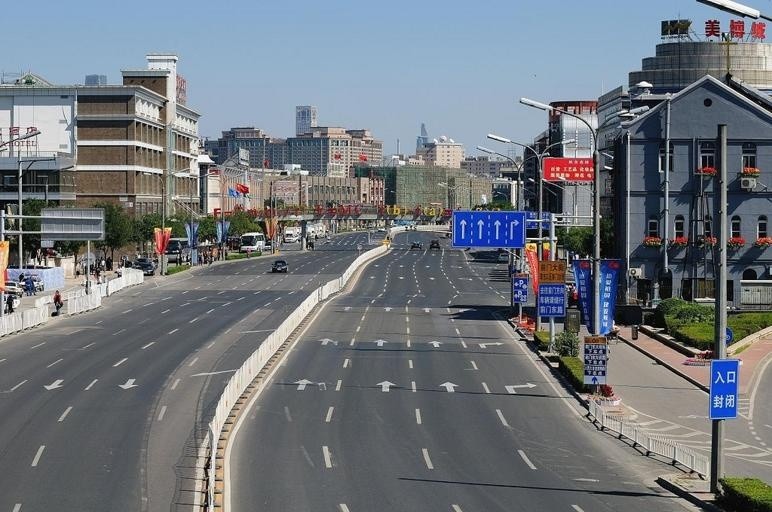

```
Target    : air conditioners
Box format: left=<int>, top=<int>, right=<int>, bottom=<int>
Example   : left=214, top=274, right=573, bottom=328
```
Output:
left=628, top=267, right=641, bottom=277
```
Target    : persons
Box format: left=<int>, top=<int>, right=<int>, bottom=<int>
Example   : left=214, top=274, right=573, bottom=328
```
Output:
left=197, top=247, right=224, bottom=267
left=7, top=295, right=14, bottom=314
left=25, top=276, right=33, bottom=296
left=246, top=248, right=251, bottom=259
left=53, top=289, right=64, bottom=316
left=19, top=272, right=25, bottom=282
left=30, top=274, right=37, bottom=295
left=75, top=256, right=122, bottom=295
left=567, top=282, right=576, bottom=292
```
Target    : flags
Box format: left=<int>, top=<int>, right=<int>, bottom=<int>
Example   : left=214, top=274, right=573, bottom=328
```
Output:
left=359, top=153, right=368, bottom=161
left=335, top=154, right=341, bottom=160
left=236, top=183, right=250, bottom=194
left=229, top=188, right=241, bottom=198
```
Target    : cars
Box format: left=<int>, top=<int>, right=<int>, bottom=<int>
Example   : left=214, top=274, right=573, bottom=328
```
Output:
left=134, top=248, right=167, bottom=277
left=496, top=252, right=509, bottom=264
left=270, top=259, right=289, bottom=274
left=411, top=241, right=423, bottom=249
left=1, top=271, right=45, bottom=314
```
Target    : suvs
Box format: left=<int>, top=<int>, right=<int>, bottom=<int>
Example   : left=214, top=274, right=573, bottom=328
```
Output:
left=430, top=239, right=441, bottom=250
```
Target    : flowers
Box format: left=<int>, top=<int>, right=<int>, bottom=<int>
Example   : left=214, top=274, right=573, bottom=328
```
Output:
left=694, top=166, right=760, bottom=174
left=641, top=235, right=772, bottom=248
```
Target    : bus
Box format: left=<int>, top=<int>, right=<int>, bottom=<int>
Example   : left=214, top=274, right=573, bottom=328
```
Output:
left=165, top=223, right=326, bottom=262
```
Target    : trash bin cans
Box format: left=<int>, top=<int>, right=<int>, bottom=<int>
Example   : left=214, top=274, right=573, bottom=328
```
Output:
left=631, top=325, right=639, bottom=341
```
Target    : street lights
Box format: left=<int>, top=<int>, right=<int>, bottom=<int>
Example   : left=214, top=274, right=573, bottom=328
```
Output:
left=699, top=0, right=771, bottom=24
left=38, top=164, right=76, bottom=208
left=487, top=130, right=578, bottom=346
left=179, top=171, right=215, bottom=266
left=516, top=96, right=650, bottom=398
left=437, top=179, right=464, bottom=216
left=142, top=167, right=191, bottom=277
left=0, top=130, right=42, bottom=153
left=208, top=172, right=244, bottom=261
left=258, top=174, right=398, bottom=255
left=473, top=142, right=549, bottom=314
